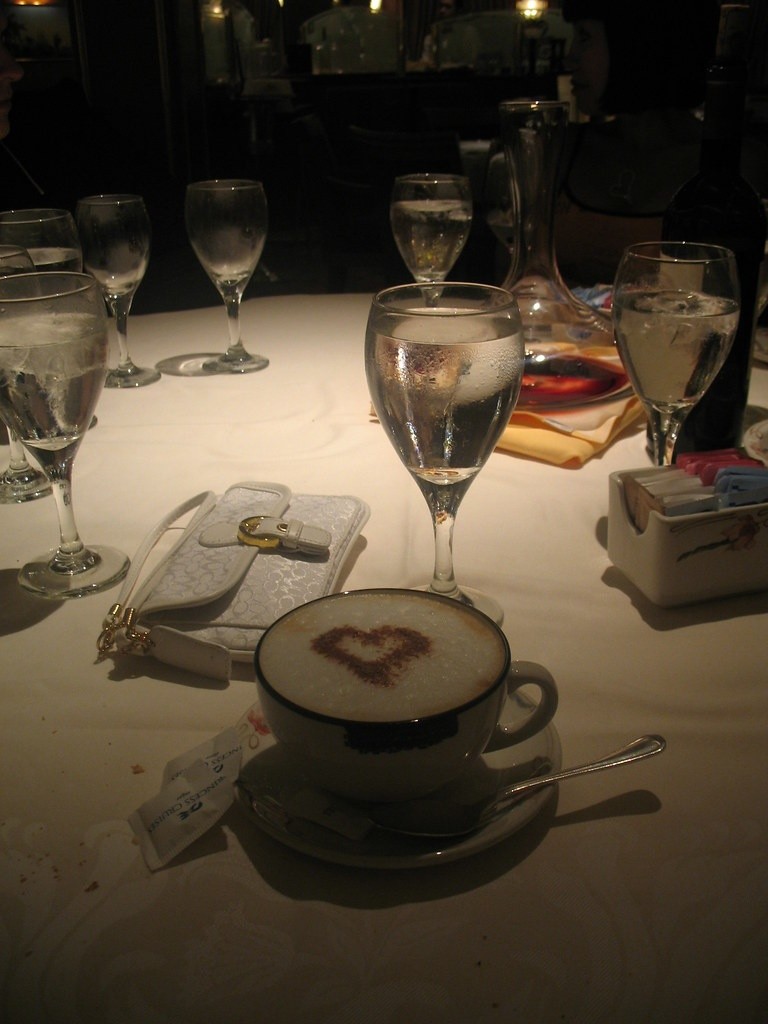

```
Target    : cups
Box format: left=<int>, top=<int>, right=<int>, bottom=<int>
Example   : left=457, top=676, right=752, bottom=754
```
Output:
left=254, top=588, right=558, bottom=804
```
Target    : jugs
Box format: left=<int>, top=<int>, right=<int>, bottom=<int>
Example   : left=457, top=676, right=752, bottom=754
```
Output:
left=484, top=100, right=632, bottom=410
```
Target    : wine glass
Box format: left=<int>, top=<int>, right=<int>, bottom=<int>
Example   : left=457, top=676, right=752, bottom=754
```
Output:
left=391, top=173, right=473, bottom=307
left=365, top=282, right=524, bottom=628
left=0, top=272, right=130, bottom=602
left=0, top=245, right=55, bottom=504
left=486, top=154, right=515, bottom=268
left=0, top=208, right=98, bottom=426
left=187, top=179, right=269, bottom=372
left=77, top=195, right=161, bottom=388
left=612, top=243, right=740, bottom=467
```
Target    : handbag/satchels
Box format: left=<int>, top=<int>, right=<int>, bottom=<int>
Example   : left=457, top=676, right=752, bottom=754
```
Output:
left=96, top=483, right=367, bottom=682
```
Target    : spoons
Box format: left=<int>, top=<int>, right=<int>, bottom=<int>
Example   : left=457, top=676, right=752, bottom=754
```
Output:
left=368, top=735, right=667, bottom=837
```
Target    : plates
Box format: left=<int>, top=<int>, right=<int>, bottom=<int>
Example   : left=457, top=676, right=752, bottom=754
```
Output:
left=235, top=689, right=561, bottom=869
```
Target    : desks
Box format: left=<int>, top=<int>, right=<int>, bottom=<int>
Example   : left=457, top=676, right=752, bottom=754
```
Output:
left=0, top=291, right=768, bottom=1024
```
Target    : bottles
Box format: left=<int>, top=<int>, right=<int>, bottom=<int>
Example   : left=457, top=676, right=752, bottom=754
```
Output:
left=661, top=3, right=768, bottom=448
